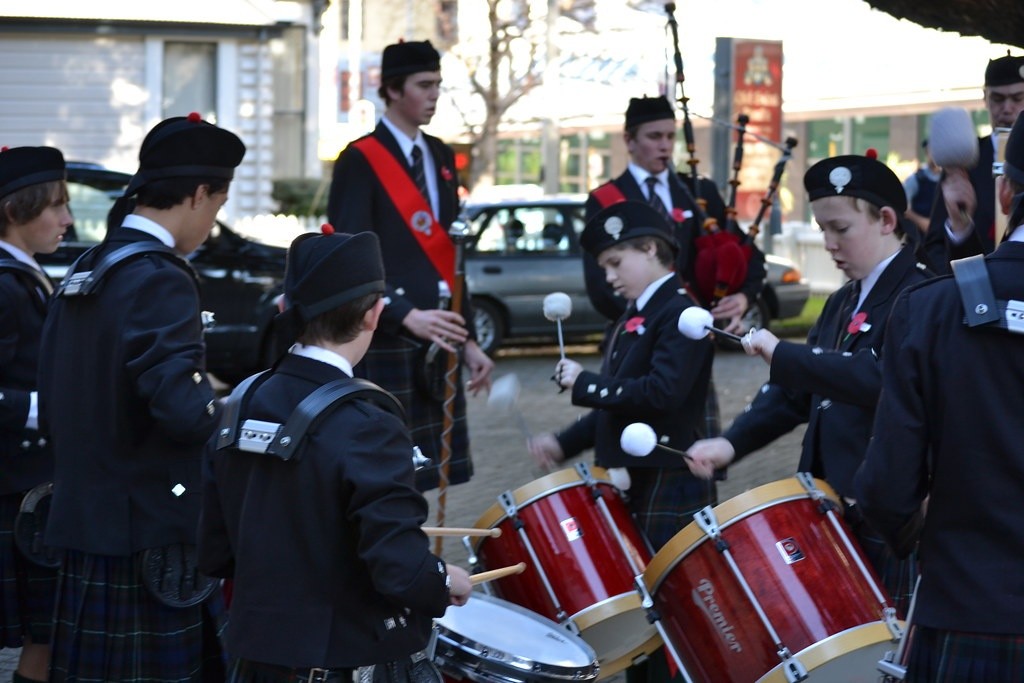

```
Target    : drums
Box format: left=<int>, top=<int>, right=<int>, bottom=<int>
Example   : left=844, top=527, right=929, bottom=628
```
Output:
left=423, top=590, right=600, bottom=683
left=633, top=471, right=904, bottom=683
left=461, top=462, right=666, bottom=683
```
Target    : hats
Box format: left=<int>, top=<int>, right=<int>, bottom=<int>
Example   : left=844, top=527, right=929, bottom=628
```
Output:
left=381, top=38, right=441, bottom=80
left=985, top=50, right=1024, bottom=86
left=805, top=149, right=907, bottom=216
left=578, top=199, right=682, bottom=259
left=0, top=146, right=66, bottom=197
left=282, top=224, right=385, bottom=320
left=625, top=95, right=675, bottom=128
left=139, top=112, right=246, bottom=178
left=1005, top=110, right=1024, bottom=170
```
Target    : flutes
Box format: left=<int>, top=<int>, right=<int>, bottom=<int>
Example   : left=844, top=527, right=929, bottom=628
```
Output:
left=664, top=1, right=799, bottom=313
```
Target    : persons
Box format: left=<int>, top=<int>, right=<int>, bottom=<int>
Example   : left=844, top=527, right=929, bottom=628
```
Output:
left=902, top=140, right=941, bottom=242
left=0, top=145, right=73, bottom=682
left=850, top=49, right=1024, bottom=683
left=325, top=39, right=495, bottom=494
left=579, top=95, right=766, bottom=481
left=36, top=111, right=249, bottom=683
left=683, top=148, right=939, bottom=623
left=196, top=222, right=472, bottom=683
left=525, top=201, right=717, bottom=683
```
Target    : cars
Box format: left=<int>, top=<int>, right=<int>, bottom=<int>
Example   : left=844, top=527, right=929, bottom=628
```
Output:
left=453, top=191, right=812, bottom=365
left=29, top=160, right=294, bottom=389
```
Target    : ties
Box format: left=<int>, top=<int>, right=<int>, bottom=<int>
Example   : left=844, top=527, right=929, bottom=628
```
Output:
left=835, top=280, right=861, bottom=351
left=645, top=176, right=673, bottom=226
left=625, top=301, right=638, bottom=322
left=411, top=144, right=433, bottom=211
